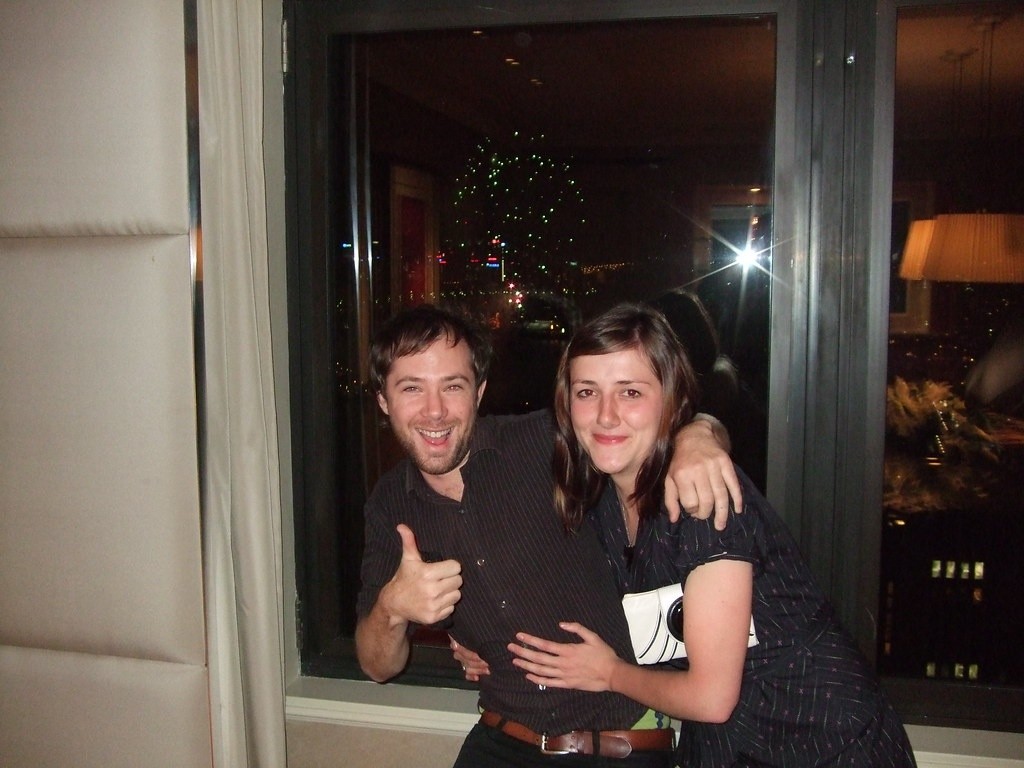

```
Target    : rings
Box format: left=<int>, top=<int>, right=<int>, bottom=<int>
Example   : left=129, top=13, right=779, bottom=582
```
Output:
left=462, top=664, right=466, bottom=672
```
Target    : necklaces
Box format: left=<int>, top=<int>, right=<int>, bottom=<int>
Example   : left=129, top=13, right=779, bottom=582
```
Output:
left=615, top=488, right=640, bottom=548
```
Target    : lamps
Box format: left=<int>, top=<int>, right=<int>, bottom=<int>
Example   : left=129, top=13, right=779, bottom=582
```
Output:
left=922, top=211, right=1024, bottom=284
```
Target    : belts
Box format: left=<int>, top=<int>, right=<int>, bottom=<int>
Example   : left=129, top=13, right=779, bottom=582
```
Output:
left=481, top=709, right=672, bottom=759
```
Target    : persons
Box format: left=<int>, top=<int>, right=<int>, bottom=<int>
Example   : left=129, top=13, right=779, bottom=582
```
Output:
left=641, top=285, right=738, bottom=406
left=446, top=305, right=918, bottom=768
left=353, top=300, right=747, bottom=767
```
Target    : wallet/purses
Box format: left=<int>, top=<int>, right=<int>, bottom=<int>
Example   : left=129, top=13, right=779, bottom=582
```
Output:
left=621, top=583, right=760, bottom=665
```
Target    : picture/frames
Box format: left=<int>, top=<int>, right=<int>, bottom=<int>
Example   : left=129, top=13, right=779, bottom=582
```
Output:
left=689, top=180, right=938, bottom=338
